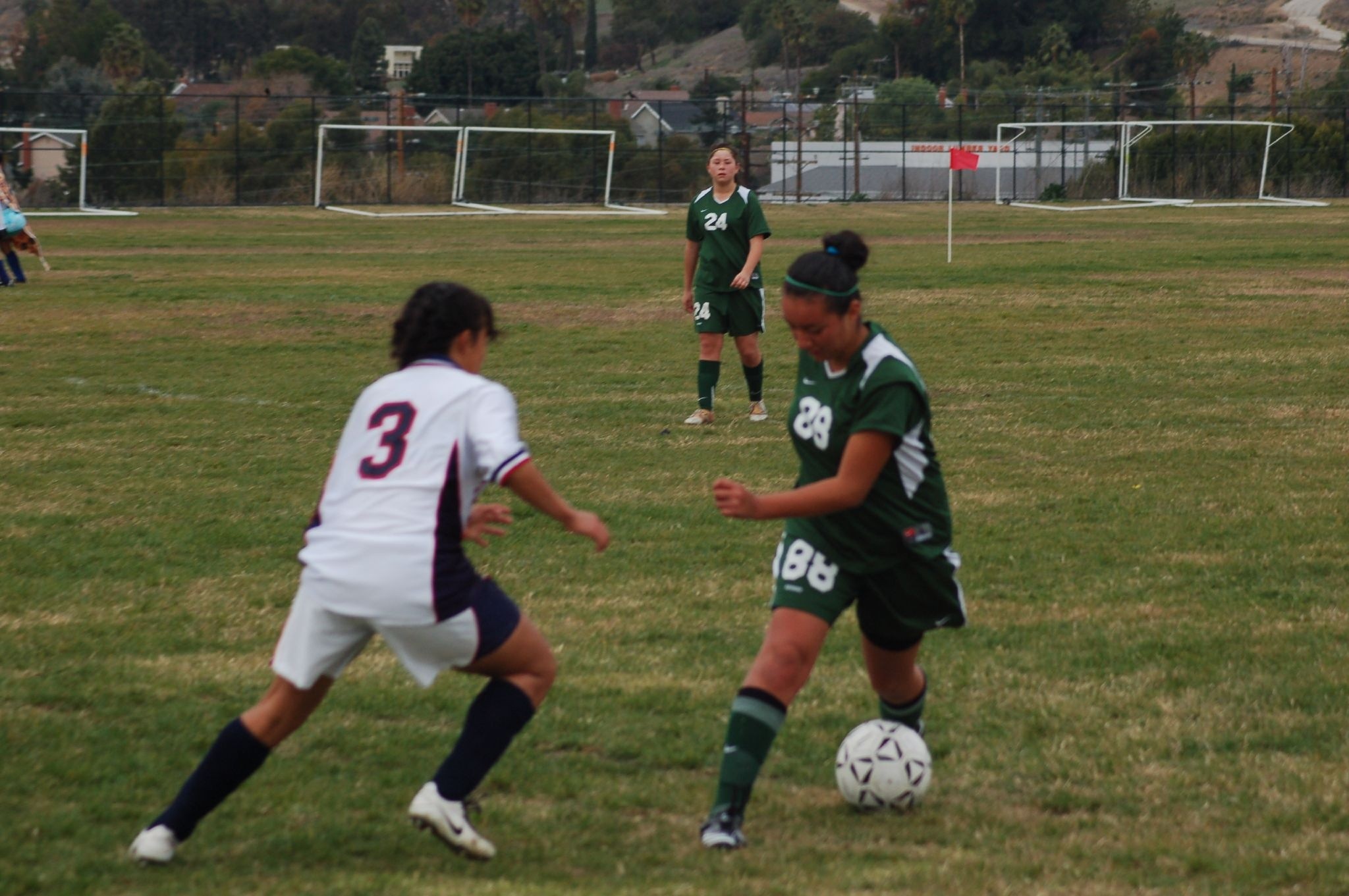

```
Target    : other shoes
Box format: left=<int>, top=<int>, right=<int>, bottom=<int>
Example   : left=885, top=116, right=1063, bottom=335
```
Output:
left=13, top=277, right=25, bottom=283
left=0, top=278, right=13, bottom=287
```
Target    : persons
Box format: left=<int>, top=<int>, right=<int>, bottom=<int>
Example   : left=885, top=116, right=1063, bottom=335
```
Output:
left=0, top=170, right=50, bottom=288
left=698, top=229, right=967, bottom=851
left=681, top=141, right=769, bottom=425
left=129, top=280, right=612, bottom=865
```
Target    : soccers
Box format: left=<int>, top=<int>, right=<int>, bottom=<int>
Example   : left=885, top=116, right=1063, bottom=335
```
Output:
left=834, top=718, right=932, bottom=808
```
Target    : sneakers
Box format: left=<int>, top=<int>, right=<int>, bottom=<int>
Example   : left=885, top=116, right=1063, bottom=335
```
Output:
left=700, top=804, right=746, bottom=847
left=749, top=397, right=768, bottom=421
left=683, top=408, right=716, bottom=425
left=409, top=784, right=505, bottom=860
left=128, top=825, right=179, bottom=862
